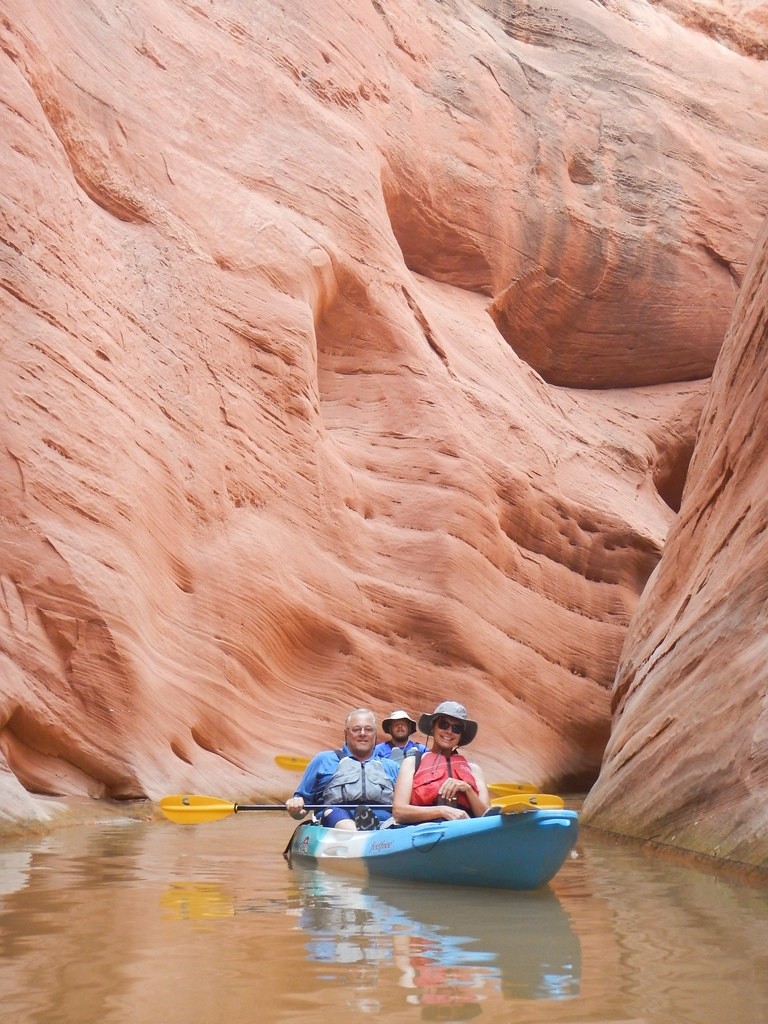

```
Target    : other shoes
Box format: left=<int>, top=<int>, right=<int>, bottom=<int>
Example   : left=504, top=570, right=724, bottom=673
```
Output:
left=354, top=805, right=380, bottom=831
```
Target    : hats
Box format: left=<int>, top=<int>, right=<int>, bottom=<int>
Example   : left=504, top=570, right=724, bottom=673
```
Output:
left=418, top=700, right=479, bottom=747
left=382, top=711, right=417, bottom=737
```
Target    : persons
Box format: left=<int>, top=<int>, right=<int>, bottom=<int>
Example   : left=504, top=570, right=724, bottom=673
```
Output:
left=375, top=710, right=429, bottom=765
left=392, top=701, right=502, bottom=826
left=285, top=708, right=401, bottom=831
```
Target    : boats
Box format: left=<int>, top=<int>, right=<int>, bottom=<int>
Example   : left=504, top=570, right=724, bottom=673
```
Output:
left=281, top=806, right=582, bottom=897
left=286, top=870, right=584, bottom=1002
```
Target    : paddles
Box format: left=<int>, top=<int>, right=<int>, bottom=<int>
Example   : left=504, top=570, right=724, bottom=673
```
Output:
left=275, top=754, right=542, bottom=798
left=160, top=794, right=566, bottom=826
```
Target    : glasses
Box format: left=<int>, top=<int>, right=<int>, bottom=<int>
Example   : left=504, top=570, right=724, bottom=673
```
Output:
left=434, top=719, right=463, bottom=734
